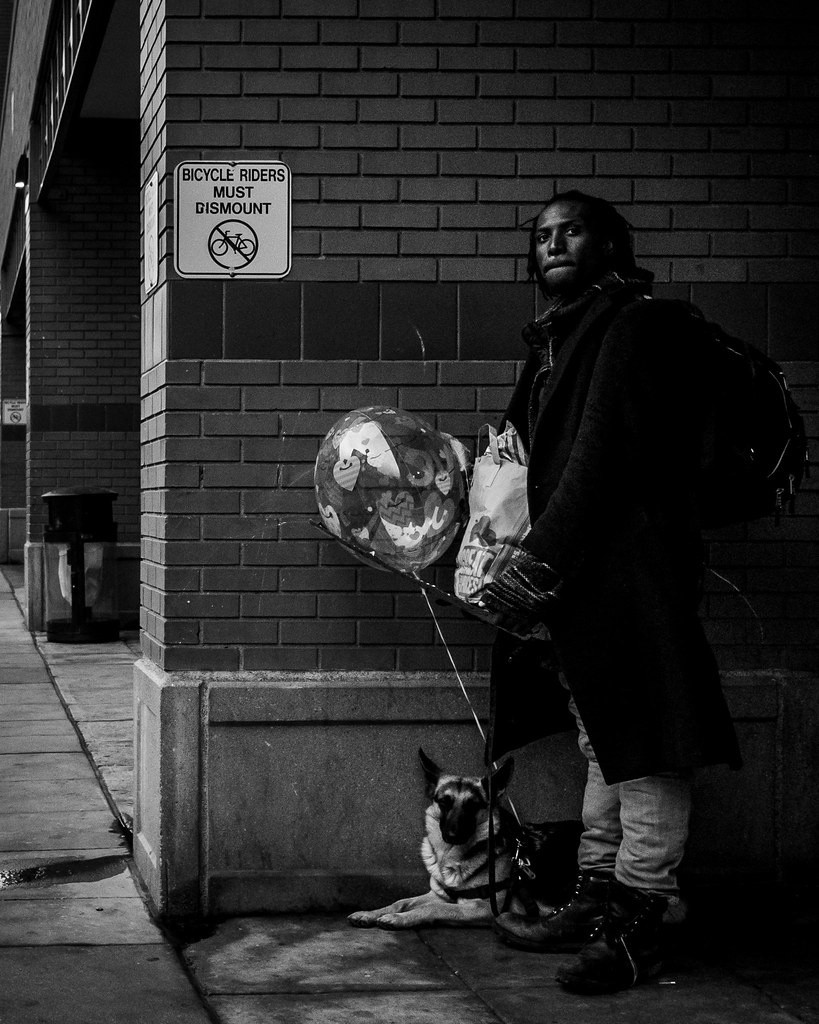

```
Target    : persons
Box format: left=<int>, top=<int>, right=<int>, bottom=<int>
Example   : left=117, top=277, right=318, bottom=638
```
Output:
left=467, top=186, right=733, bottom=994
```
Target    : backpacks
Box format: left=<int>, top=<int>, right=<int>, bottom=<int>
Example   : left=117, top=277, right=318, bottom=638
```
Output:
left=687, top=331, right=810, bottom=543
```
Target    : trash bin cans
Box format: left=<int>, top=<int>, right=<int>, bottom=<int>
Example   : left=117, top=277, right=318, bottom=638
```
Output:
left=43, top=488, right=123, bottom=644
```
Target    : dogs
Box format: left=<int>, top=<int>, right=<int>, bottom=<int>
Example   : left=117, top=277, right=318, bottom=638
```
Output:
left=347, top=746, right=586, bottom=930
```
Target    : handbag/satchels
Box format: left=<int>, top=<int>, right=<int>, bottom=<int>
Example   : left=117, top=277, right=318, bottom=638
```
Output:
left=453, top=420, right=553, bottom=641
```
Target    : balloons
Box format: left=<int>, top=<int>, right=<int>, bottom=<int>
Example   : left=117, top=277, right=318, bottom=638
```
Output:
left=311, top=402, right=457, bottom=580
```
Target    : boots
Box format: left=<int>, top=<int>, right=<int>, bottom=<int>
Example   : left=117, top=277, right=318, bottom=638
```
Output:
left=555, top=878, right=669, bottom=993
left=492, top=870, right=612, bottom=952
